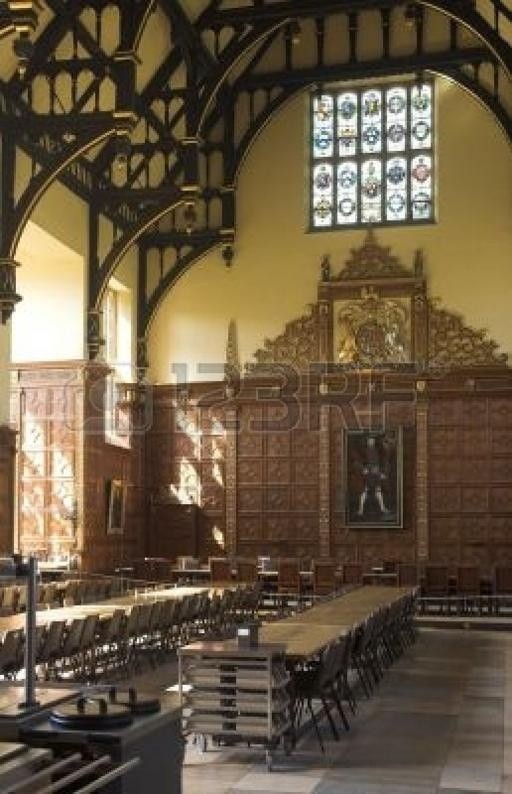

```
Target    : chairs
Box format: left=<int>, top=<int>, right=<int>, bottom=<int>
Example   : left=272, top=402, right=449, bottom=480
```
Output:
left=1, top=554, right=510, bottom=757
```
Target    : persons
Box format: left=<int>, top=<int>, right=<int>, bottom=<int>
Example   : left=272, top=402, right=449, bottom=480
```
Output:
left=353, top=433, right=394, bottom=517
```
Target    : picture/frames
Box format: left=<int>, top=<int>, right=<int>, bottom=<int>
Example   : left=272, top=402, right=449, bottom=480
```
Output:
left=342, top=425, right=404, bottom=529
left=107, top=480, right=128, bottom=534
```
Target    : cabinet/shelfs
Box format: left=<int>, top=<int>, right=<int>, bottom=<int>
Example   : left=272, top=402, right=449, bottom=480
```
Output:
left=176, top=639, right=290, bottom=771
